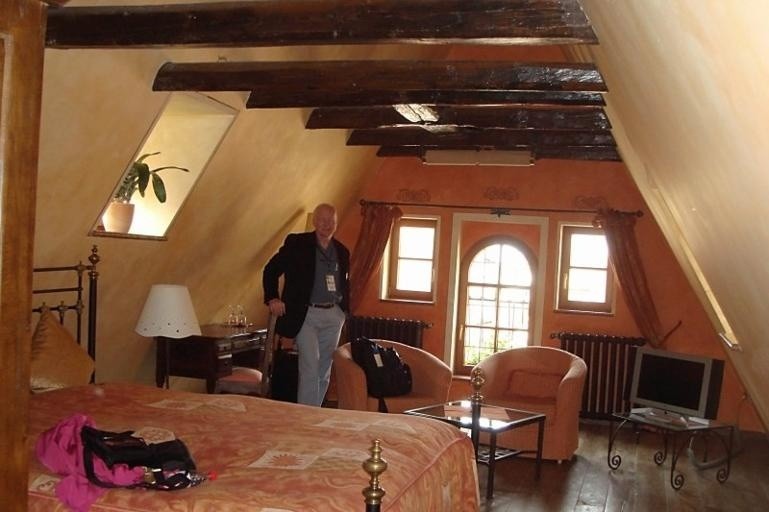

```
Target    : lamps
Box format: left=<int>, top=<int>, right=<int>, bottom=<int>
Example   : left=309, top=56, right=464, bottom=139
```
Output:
left=133, top=285, right=203, bottom=389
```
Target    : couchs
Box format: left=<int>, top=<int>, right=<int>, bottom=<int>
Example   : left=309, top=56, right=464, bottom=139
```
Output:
left=332, top=338, right=454, bottom=414
left=469, top=346, right=588, bottom=461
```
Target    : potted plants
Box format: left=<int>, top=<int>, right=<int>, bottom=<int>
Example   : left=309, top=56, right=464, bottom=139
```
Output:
left=99, top=150, right=189, bottom=233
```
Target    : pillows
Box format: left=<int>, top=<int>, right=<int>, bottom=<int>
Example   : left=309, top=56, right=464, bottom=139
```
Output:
left=27, top=302, right=95, bottom=392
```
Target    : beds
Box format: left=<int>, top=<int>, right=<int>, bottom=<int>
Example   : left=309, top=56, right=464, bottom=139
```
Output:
left=26, top=246, right=483, bottom=512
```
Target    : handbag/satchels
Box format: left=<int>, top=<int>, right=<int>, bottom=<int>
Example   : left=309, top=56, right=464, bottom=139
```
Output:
left=81, top=425, right=195, bottom=491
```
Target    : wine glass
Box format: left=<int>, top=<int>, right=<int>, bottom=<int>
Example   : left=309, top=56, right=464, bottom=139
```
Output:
left=227, top=305, right=238, bottom=324
left=236, top=304, right=247, bottom=326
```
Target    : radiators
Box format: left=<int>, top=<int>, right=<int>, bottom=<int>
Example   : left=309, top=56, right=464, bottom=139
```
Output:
left=342, top=315, right=430, bottom=348
left=550, top=331, right=647, bottom=422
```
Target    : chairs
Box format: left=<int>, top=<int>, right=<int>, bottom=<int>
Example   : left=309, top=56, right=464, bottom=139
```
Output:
left=213, top=310, right=278, bottom=398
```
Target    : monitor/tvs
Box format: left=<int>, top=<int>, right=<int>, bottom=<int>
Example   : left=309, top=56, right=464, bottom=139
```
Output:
left=629, top=344, right=725, bottom=421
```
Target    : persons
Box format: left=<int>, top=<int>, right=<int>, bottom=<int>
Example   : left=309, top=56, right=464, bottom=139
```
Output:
left=262, top=204, right=353, bottom=407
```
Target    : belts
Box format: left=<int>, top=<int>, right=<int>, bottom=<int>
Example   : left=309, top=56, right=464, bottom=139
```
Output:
left=309, top=303, right=334, bottom=308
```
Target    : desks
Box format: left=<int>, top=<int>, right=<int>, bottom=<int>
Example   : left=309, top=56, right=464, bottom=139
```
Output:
left=608, top=408, right=735, bottom=490
left=155, top=319, right=268, bottom=392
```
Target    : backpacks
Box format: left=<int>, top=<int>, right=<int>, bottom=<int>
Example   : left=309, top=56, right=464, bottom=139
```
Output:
left=352, top=337, right=412, bottom=400
